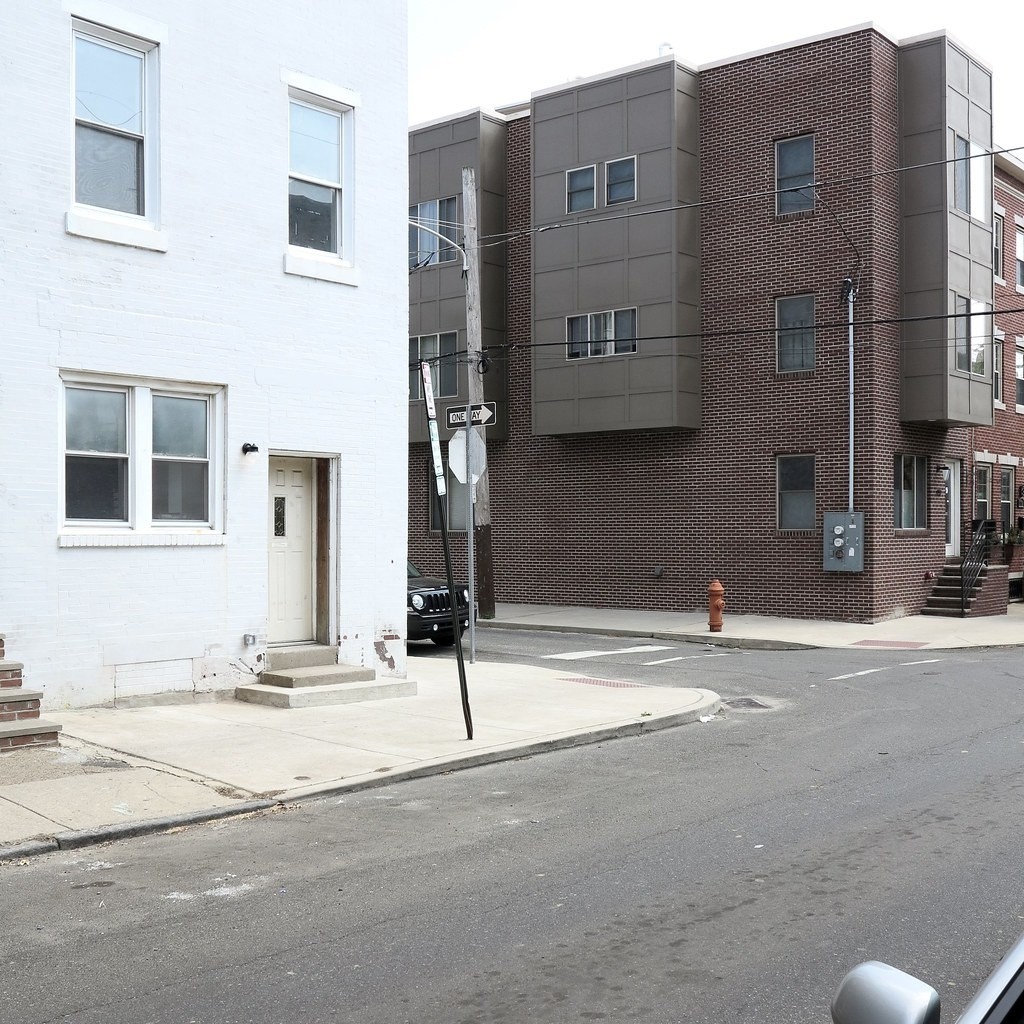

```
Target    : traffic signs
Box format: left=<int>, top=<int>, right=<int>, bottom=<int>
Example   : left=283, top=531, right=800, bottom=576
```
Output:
left=445, top=401, right=497, bottom=430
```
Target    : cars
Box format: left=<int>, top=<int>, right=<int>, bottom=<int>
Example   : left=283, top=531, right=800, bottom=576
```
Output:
left=826, top=925, right=1024, bottom=1024
left=407, top=559, right=471, bottom=647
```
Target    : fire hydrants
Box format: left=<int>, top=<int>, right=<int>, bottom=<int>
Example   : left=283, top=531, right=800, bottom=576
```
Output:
left=708, top=579, right=726, bottom=633
